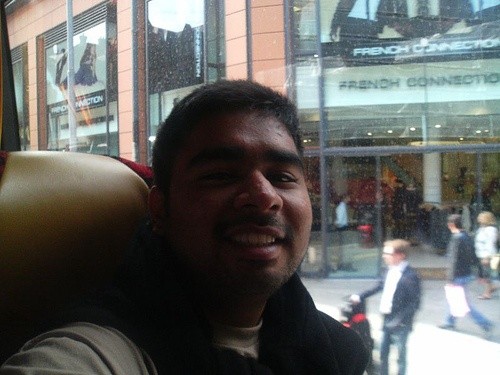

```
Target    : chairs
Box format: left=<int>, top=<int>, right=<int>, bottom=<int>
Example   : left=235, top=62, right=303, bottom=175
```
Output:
left=0, top=150, right=150, bottom=370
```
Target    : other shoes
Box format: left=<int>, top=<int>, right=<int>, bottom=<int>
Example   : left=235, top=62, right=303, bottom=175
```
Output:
left=478, top=294, right=489, bottom=299
left=439, top=323, right=453, bottom=329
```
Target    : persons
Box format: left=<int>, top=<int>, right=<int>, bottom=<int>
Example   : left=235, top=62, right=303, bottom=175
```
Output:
left=350, top=239, right=422, bottom=374
left=473, top=210, right=498, bottom=298
left=331, top=178, right=408, bottom=240
left=0, top=79, right=369, bottom=375
left=441, top=213, right=493, bottom=338
